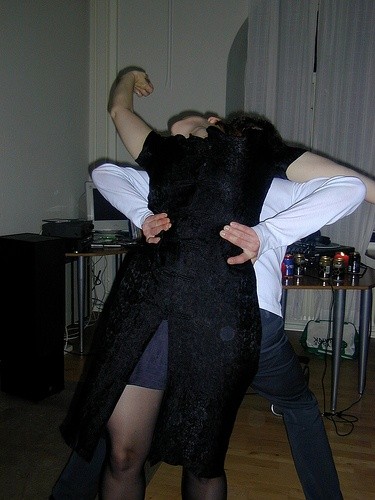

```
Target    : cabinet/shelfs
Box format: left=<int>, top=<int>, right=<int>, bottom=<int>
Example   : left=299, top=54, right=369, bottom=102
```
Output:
left=0, top=233, right=65, bottom=404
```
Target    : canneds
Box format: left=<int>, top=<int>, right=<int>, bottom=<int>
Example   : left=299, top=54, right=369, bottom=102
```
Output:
left=319, top=256, right=330, bottom=280
left=293, top=253, right=305, bottom=277
left=348, top=251, right=361, bottom=273
left=281, top=254, right=294, bottom=277
left=332, top=259, right=343, bottom=281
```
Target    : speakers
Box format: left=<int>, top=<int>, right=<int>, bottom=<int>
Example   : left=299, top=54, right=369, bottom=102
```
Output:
left=1, top=233, right=65, bottom=403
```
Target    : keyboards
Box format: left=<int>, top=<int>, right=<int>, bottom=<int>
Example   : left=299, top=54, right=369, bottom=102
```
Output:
left=91, top=241, right=123, bottom=248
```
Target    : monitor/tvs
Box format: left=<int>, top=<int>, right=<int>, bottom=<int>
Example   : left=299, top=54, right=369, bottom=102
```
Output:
left=84, top=181, right=129, bottom=234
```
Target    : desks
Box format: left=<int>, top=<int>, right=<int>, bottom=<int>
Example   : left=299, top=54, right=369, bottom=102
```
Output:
left=283, top=264, right=375, bottom=415
left=65, top=248, right=128, bottom=356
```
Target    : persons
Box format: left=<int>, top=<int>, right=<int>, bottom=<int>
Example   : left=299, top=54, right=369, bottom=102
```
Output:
left=58, top=70, right=375, bottom=500
left=49, top=162, right=366, bottom=500
